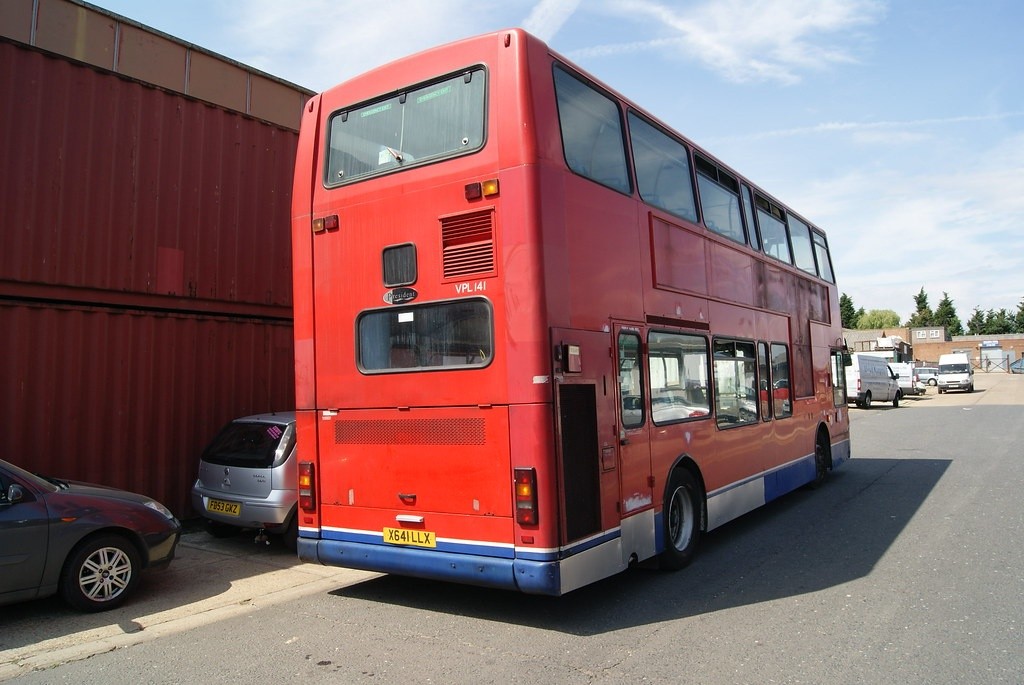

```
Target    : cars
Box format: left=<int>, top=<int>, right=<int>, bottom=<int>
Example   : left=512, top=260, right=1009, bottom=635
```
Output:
left=0, top=458, right=182, bottom=613
left=192, top=409, right=299, bottom=553
left=622, top=395, right=710, bottom=426
left=914, top=367, right=939, bottom=387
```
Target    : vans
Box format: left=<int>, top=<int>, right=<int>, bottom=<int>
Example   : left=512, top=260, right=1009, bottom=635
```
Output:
left=831, top=354, right=903, bottom=407
left=937, top=354, right=974, bottom=394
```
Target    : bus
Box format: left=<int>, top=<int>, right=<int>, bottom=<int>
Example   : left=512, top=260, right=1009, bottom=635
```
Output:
left=291, top=48, right=852, bottom=600
